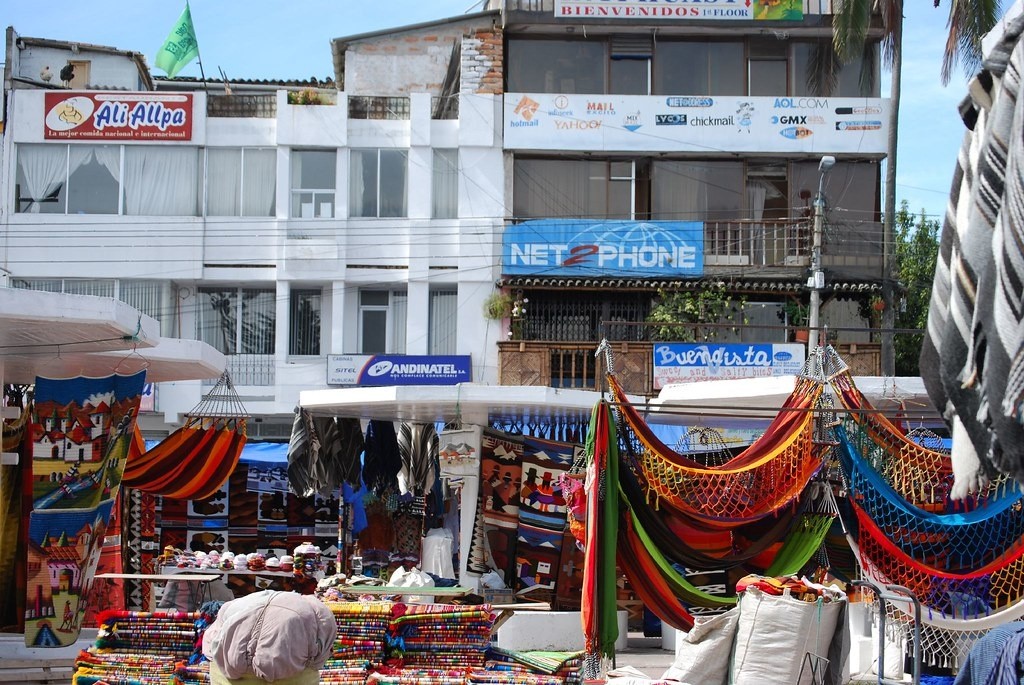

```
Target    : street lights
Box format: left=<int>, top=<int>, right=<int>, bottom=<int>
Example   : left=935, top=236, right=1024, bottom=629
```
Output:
left=806, top=156, right=839, bottom=376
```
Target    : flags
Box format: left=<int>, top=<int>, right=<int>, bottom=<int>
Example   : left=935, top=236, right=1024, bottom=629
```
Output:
left=155, top=6, right=199, bottom=78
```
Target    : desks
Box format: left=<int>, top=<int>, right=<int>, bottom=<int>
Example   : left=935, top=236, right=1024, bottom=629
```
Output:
left=94, top=573, right=220, bottom=613
left=175, top=568, right=296, bottom=612
left=343, top=587, right=474, bottom=597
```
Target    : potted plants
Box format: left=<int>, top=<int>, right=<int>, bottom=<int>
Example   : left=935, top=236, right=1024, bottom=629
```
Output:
left=783, top=296, right=808, bottom=343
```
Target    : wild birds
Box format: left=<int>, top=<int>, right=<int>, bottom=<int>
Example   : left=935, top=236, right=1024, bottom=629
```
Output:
left=40, top=65, right=54, bottom=84
left=60, top=63, right=75, bottom=89
